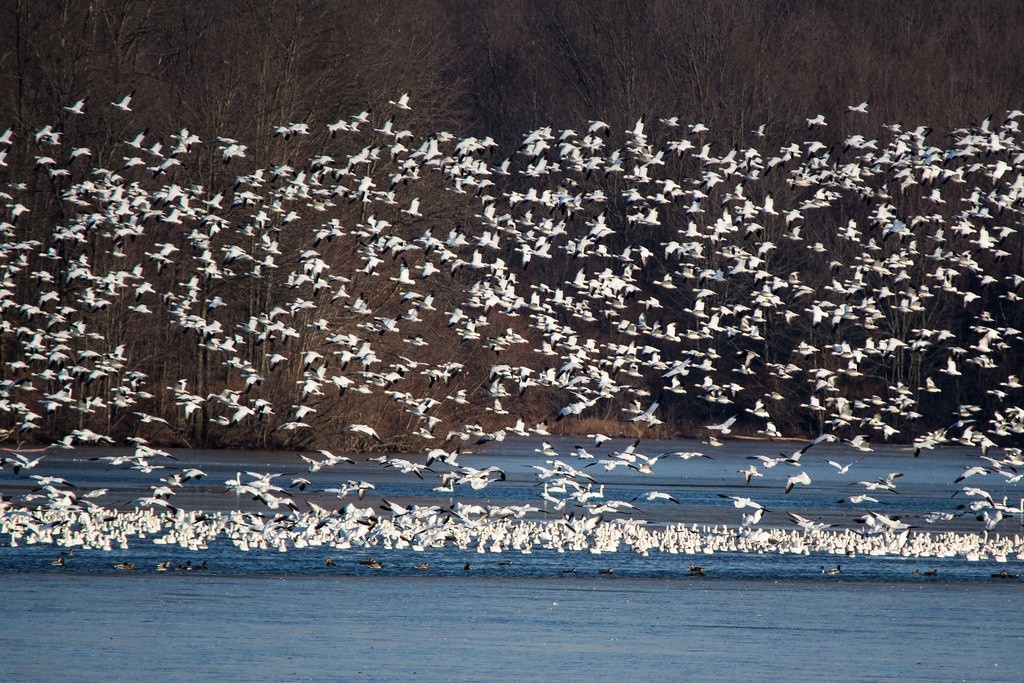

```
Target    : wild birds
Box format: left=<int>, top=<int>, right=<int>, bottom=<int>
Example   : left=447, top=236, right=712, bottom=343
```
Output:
left=0, top=88, right=1023, bottom=582
